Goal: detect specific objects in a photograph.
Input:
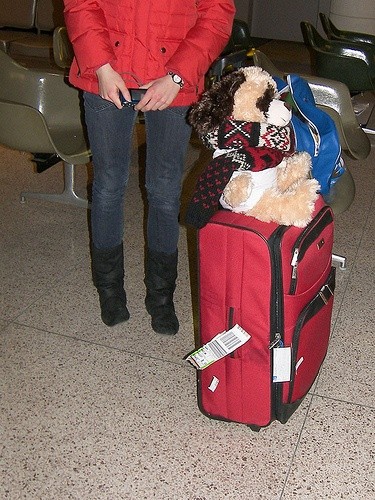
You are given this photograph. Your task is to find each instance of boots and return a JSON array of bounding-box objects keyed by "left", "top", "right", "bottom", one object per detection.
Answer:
[
  {"left": 89, "top": 243, "right": 129, "bottom": 327},
  {"left": 144, "top": 245, "right": 178, "bottom": 334}
]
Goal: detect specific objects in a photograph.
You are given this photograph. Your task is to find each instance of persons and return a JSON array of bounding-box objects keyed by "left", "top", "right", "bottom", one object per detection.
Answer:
[{"left": 61, "top": 0, "right": 238, "bottom": 336}]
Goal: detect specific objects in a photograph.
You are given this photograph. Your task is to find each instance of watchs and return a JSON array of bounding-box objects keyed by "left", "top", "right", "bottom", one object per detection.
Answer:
[{"left": 167, "top": 71, "right": 184, "bottom": 89}]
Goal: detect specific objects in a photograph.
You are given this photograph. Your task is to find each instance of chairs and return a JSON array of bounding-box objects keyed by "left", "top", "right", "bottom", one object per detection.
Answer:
[
  {"left": 8, "top": 0, "right": 64, "bottom": 59},
  {"left": 231, "top": 19, "right": 254, "bottom": 49},
  {"left": 319, "top": 12, "right": 375, "bottom": 51},
  {"left": 300, "top": 20, "right": 374, "bottom": 92},
  {"left": 0, "top": 47, "right": 93, "bottom": 210},
  {"left": 0, "top": 0, "right": 36, "bottom": 52},
  {"left": 328, "top": 161, "right": 357, "bottom": 272},
  {"left": 252, "top": 50, "right": 375, "bottom": 161}
]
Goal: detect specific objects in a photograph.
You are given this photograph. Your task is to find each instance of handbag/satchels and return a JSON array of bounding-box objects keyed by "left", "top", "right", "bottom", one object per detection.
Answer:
[{"left": 270, "top": 74, "right": 345, "bottom": 196}]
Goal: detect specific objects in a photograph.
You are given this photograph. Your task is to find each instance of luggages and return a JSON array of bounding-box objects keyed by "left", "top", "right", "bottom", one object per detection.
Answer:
[{"left": 188, "top": 48, "right": 335, "bottom": 433}]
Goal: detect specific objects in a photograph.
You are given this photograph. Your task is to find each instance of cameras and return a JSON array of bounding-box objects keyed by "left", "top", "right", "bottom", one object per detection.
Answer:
[{"left": 119, "top": 88, "right": 151, "bottom": 108}]
